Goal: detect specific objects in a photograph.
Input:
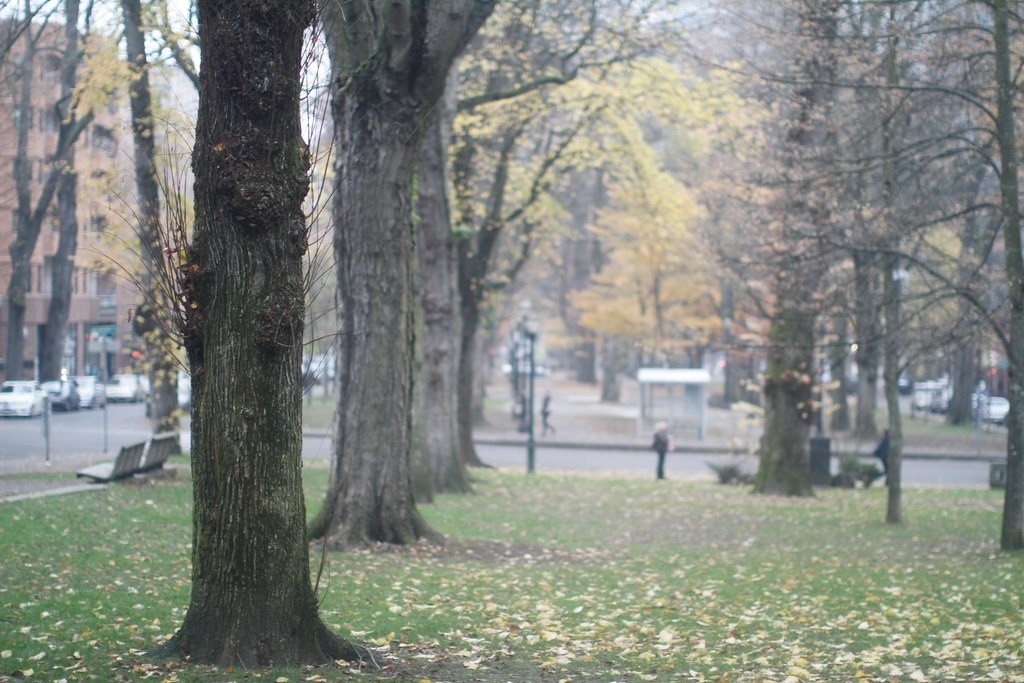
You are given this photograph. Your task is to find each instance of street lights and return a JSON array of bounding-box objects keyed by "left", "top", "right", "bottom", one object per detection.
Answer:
[{"left": 520, "top": 318, "right": 542, "bottom": 472}]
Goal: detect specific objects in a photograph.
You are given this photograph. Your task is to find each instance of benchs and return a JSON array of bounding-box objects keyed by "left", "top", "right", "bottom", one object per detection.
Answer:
[
  {"left": 136, "top": 434, "right": 176, "bottom": 473},
  {"left": 76, "top": 441, "right": 146, "bottom": 483}
]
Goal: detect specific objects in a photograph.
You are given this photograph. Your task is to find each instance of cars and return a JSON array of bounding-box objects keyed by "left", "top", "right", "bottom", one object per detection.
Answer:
[
  {"left": 0, "top": 380, "right": 50, "bottom": 417},
  {"left": 42, "top": 380, "right": 81, "bottom": 412},
  {"left": 105, "top": 373, "right": 146, "bottom": 404},
  {"left": 68, "top": 375, "right": 108, "bottom": 409},
  {"left": 898, "top": 374, "right": 1010, "bottom": 424}
]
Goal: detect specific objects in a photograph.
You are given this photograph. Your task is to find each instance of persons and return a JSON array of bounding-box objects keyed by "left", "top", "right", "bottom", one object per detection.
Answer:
[
  {"left": 541, "top": 392, "right": 556, "bottom": 437},
  {"left": 652, "top": 422, "right": 669, "bottom": 478},
  {"left": 873, "top": 429, "right": 890, "bottom": 484}
]
